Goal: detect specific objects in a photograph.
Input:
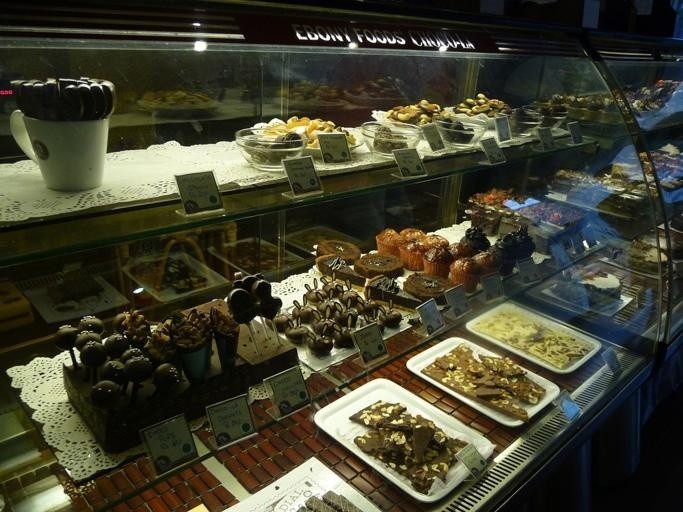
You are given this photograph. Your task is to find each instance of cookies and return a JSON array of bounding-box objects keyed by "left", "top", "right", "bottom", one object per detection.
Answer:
[
  {"left": 295, "top": 490, "right": 363, "bottom": 512},
  {"left": 257, "top": 91, "right": 516, "bottom": 147},
  {"left": 349, "top": 400, "right": 467, "bottom": 494},
  {"left": 136, "top": 76, "right": 418, "bottom": 108}
]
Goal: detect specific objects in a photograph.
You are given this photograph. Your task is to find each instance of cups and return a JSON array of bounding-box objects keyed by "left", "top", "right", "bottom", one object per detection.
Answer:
[{"left": 7, "top": 110, "right": 110, "bottom": 192}]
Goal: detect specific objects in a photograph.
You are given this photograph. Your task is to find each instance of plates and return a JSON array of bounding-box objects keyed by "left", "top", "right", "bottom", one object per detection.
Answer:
[
  {"left": 121, "top": 251, "right": 231, "bottom": 306},
  {"left": 279, "top": 226, "right": 365, "bottom": 266},
  {"left": 311, "top": 125, "right": 362, "bottom": 157},
  {"left": 381, "top": 104, "right": 508, "bottom": 129},
  {"left": 276, "top": 90, "right": 419, "bottom": 111},
  {"left": 463, "top": 301, "right": 601, "bottom": 375},
  {"left": 407, "top": 336, "right": 561, "bottom": 429},
  {"left": 136, "top": 101, "right": 224, "bottom": 116},
  {"left": 30, "top": 275, "right": 129, "bottom": 322},
  {"left": 208, "top": 237, "right": 308, "bottom": 283},
  {"left": 313, "top": 378, "right": 493, "bottom": 507}
]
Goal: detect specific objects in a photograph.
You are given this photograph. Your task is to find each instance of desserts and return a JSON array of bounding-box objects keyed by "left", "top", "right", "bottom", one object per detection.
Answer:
[{"left": 57, "top": 145, "right": 682, "bottom": 454}]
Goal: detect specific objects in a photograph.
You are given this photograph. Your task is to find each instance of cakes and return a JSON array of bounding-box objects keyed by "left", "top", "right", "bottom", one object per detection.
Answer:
[
  {"left": 0, "top": 280, "right": 35, "bottom": 336},
  {"left": 535, "top": 77, "right": 682, "bottom": 115}
]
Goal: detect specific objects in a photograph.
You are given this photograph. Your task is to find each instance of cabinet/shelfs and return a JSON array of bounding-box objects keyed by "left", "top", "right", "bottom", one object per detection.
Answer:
[{"left": 0, "top": 0, "right": 683, "bottom": 512}]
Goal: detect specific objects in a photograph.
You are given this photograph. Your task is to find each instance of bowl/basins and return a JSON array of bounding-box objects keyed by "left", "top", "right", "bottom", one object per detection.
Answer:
[
  {"left": 437, "top": 119, "right": 488, "bottom": 148},
  {"left": 234, "top": 128, "right": 308, "bottom": 175},
  {"left": 501, "top": 106, "right": 569, "bottom": 136},
  {"left": 362, "top": 123, "right": 422, "bottom": 157}
]
